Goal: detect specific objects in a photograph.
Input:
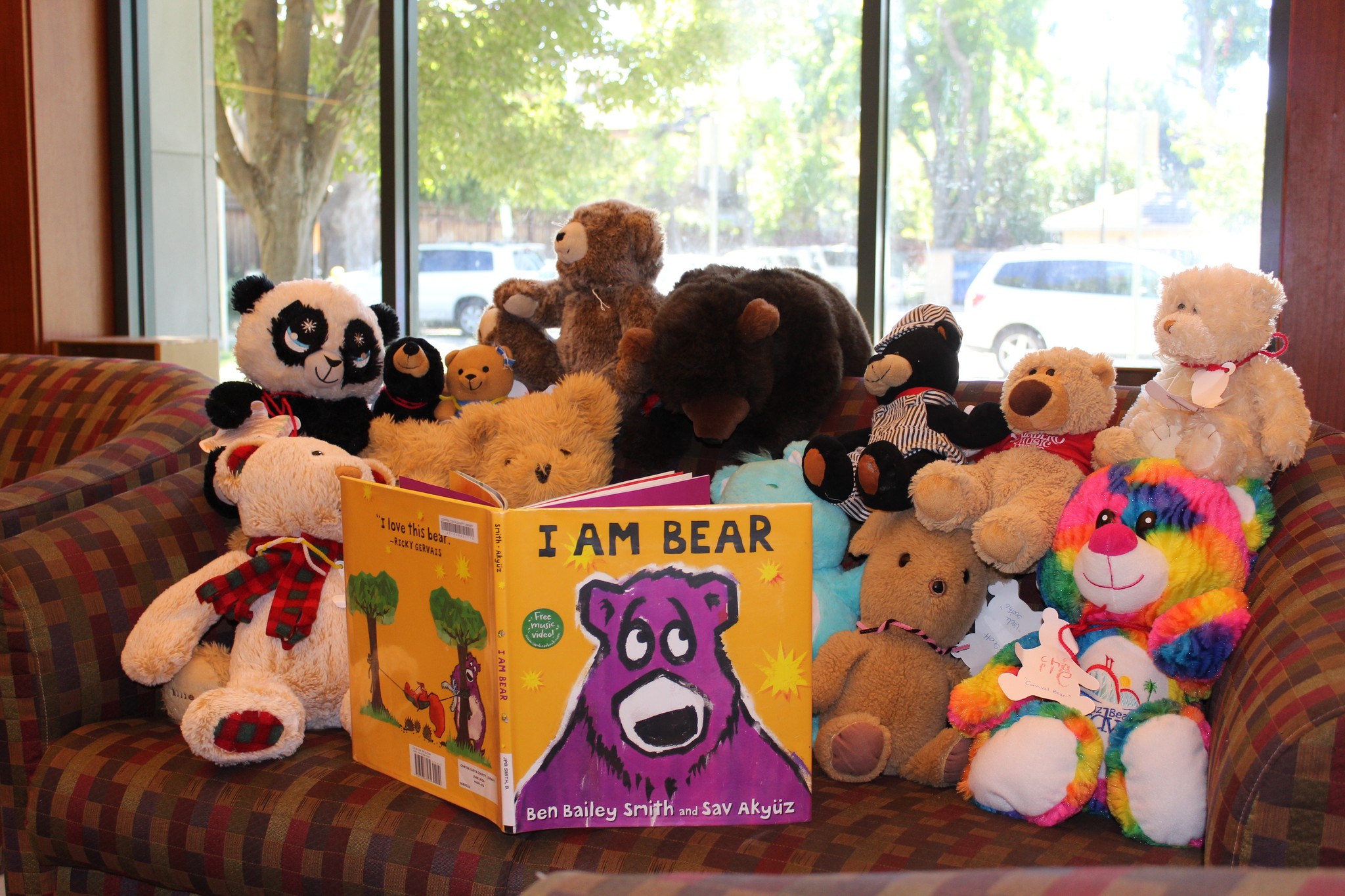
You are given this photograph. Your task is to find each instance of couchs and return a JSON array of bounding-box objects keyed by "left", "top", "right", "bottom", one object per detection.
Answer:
[{"left": 0, "top": 354, "right": 1345, "bottom": 896}]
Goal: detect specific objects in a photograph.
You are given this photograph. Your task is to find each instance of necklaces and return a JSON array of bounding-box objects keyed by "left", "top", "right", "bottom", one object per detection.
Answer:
[{"left": 1180, "top": 331, "right": 1288, "bottom": 406}]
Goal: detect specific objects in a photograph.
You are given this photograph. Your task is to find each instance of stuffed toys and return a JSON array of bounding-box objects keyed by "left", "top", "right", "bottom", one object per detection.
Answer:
[
  {"left": 907, "top": 347, "right": 1118, "bottom": 573},
  {"left": 201, "top": 275, "right": 399, "bottom": 528},
  {"left": 119, "top": 435, "right": 391, "bottom": 764},
  {"left": 1090, "top": 261, "right": 1312, "bottom": 486},
  {"left": 947, "top": 457, "right": 1278, "bottom": 846},
  {"left": 801, "top": 303, "right": 1012, "bottom": 521},
  {"left": 371, "top": 336, "right": 447, "bottom": 424},
  {"left": 434, "top": 345, "right": 514, "bottom": 425},
  {"left": 337, "top": 473, "right": 814, "bottom": 832},
  {"left": 811, "top": 508, "right": 1019, "bottom": 789},
  {"left": 608, "top": 266, "right": 876, "bottom": 486},
  {"left": 479, "top": 204, "right": 666, "bottom": 397},
  {"left": 366, "top": 369, "right": 626, "bottom": 509}
]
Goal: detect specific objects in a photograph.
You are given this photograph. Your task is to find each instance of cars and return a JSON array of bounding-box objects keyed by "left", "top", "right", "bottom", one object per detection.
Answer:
[
  {"left": 721, "top": 241, "right": 858, "bottom": 309},
  {"left": 961, "top": 252, "right": 1180, "bottom": 385}
]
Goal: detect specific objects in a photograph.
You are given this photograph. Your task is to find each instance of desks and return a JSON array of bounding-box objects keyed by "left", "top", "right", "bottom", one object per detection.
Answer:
[{"left": 51, "top": 336, "right": 219, "bottom": 382}]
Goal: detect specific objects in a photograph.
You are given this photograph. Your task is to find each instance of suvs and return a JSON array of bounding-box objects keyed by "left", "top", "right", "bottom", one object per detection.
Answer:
[{"left": 329, "top": 241, "right": 553, "bottom": 338}]
